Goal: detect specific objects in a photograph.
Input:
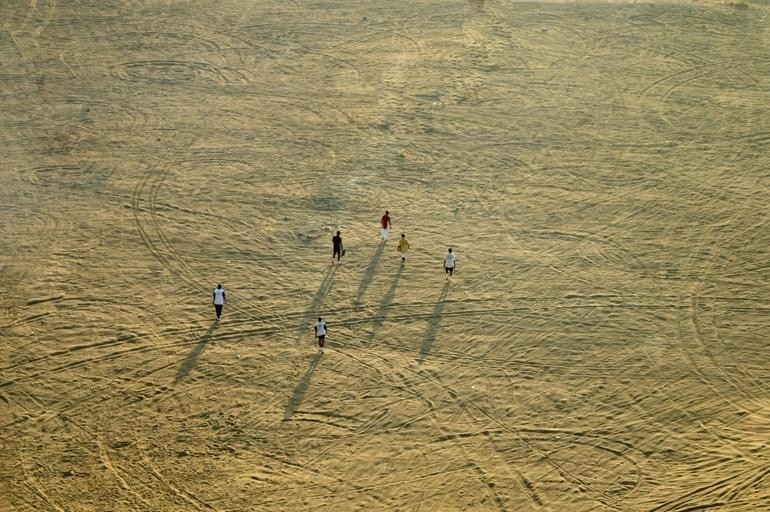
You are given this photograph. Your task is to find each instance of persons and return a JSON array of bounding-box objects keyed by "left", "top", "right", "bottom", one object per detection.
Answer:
[
  {"left": 331, "top": 230, "right": 343, "bottom": 265},
  {"left": 397, "top": 233, "right": 409, "bottom": 267},
  {"left": 443, "top": 247, "right": 456, "bottom": 283},
  {"left": 380, "top": 210, "right": 392, "bottom": 241},
  {"left": 314, "top": 316, "right": 328, "bottom": 354},
  {"left": 212, "top": 284, "right": 227, "bottom": 320}
]
